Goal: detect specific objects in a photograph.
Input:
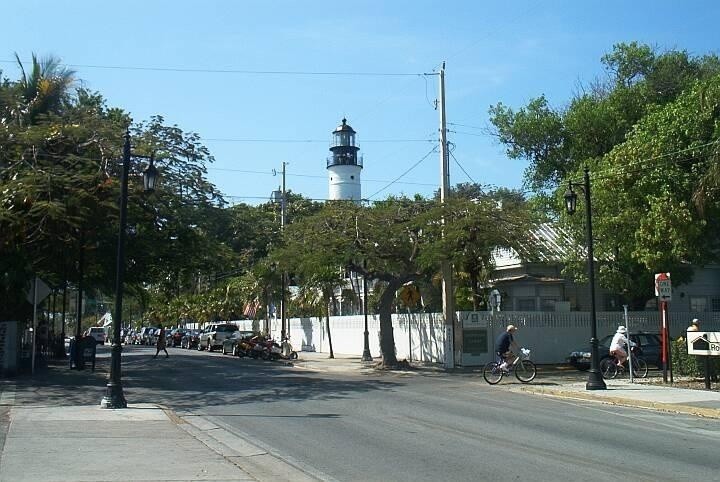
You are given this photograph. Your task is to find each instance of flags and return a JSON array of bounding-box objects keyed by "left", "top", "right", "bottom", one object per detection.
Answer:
[{"left": 243, "top": 298, "right": 258, "bottom": 321}]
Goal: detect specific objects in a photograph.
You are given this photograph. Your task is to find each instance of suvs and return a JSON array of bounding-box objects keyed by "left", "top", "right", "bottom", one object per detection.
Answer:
[{"left": 197, "top": 324, "right": 239, "bottom": 351}]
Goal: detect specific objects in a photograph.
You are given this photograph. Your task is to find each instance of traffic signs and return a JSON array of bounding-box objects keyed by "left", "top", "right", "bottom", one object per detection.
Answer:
[{"left": 655, "top": 273, "right": 670, "bottom": 296}]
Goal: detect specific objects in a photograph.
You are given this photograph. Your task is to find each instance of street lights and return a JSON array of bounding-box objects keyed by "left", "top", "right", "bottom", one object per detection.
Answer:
[
  {"left": 564, "top": 163, "right": 607, "bottom": 390},
  {"left": 101, "top": 127, "right": 160, "bottom": 409}
]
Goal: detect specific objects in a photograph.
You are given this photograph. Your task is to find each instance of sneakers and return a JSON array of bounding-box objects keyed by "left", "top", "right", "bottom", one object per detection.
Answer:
[
  {"left": 499, "top": 364, "right": 509, "bottom": 373},
  {"left": 617, "top": 363, "right": 624, "bottom": 367}
]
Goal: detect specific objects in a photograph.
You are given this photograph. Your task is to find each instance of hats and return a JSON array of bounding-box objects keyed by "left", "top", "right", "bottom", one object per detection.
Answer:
[
  {"left": 507, "top": 325, "right": 518, "bottom": 332},
  {"left": 693, "top": 319, "right": 702, "bottom": 325},
  {"left": 616, "top": 326, "right": 627, "bottom": 333}
]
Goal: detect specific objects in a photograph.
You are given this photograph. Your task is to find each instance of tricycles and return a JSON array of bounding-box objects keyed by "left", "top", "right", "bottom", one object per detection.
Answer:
[{"left": 70, "top": 337, "right": 96, "bottom": 370}]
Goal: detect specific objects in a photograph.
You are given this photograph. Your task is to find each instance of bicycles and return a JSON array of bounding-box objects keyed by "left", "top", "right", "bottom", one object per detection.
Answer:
[
  {"left": 599, "top": 345, "right": 648, "bottom": 379},
  {"left": 483, "top": 348, "right": 536, "bottom": 384}
]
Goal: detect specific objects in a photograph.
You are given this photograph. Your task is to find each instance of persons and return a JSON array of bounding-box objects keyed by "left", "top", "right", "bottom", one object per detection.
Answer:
[
  {"left": 153, "top": 324, "right": 170, "bottom": 359},
  {"left": 494, "top": 324, "right": 525, "bottom": 372},
  {"left": 610, "top": 326, "right": 639, "bottom": 367},
  {"left": 687, "top": 318, "right": 702, "bottom": 331}
]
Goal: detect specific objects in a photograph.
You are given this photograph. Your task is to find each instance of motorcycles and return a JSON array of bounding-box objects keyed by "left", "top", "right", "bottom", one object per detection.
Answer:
[{"left": 237, "top": 333, "right": 298, "bottom": 360}]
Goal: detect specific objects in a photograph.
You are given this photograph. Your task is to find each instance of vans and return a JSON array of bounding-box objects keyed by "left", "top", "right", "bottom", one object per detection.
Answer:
[{"left": 87, "top": 327, "right": 105, "bottom": 345}]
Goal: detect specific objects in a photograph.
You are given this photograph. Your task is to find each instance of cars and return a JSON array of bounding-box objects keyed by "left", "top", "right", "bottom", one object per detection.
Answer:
[
  {"left": 222, "top": 331, "right": 261, "bottom": 356},
  {"left": 570, "top": 331, "right": 675, "bottom": 371},
  {"left": 120, "top": 327, "right": 202, "bottom": 350}
]
